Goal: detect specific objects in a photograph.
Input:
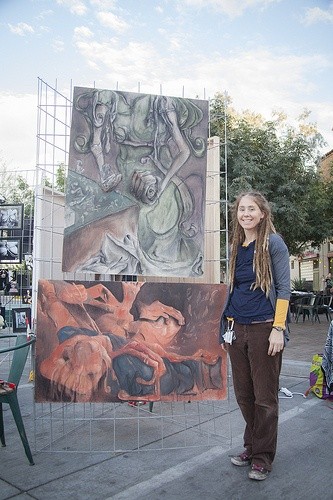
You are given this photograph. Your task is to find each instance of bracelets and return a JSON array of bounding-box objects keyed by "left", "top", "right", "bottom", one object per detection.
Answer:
[{"left": 272, "top": 326, "right": 283, "bottom": 331}]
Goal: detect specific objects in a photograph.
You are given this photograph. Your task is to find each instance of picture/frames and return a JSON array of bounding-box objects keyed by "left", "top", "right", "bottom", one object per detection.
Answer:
[
  {"left": 0, "top": 203, "right": 24, "bottom": 230},
  {"left": 0, "top": 236, "right": 23, "bottom": 264},
  {"left": 12, "top": 307, "right": 31, "bottom": 333}
]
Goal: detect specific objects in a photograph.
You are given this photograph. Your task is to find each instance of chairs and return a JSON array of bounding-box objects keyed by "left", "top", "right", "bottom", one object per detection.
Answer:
[
  {"left": 295, "top": 293, "right": 333, "bottom": 325},
  {"left": 0, "top": 333, "right": 36, "bottom": 465}
]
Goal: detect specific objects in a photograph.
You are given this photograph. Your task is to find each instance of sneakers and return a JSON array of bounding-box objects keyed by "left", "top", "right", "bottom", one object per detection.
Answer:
[
  {"left": 231, "top": 451, "right": 249, "bottom": 466},
  {"left": 249, "top": 463, "right": 268, "bottom": 480}
]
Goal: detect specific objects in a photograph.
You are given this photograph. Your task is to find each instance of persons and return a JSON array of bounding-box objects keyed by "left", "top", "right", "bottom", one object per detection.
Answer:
[{"left": 219, "top": 191, "right": 292, "bottom": 481}]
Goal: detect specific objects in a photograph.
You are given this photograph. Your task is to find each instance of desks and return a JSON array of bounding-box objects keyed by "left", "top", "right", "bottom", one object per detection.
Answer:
[{"left": 290, "top": 291, "right": 314, "bottom": 323}]
